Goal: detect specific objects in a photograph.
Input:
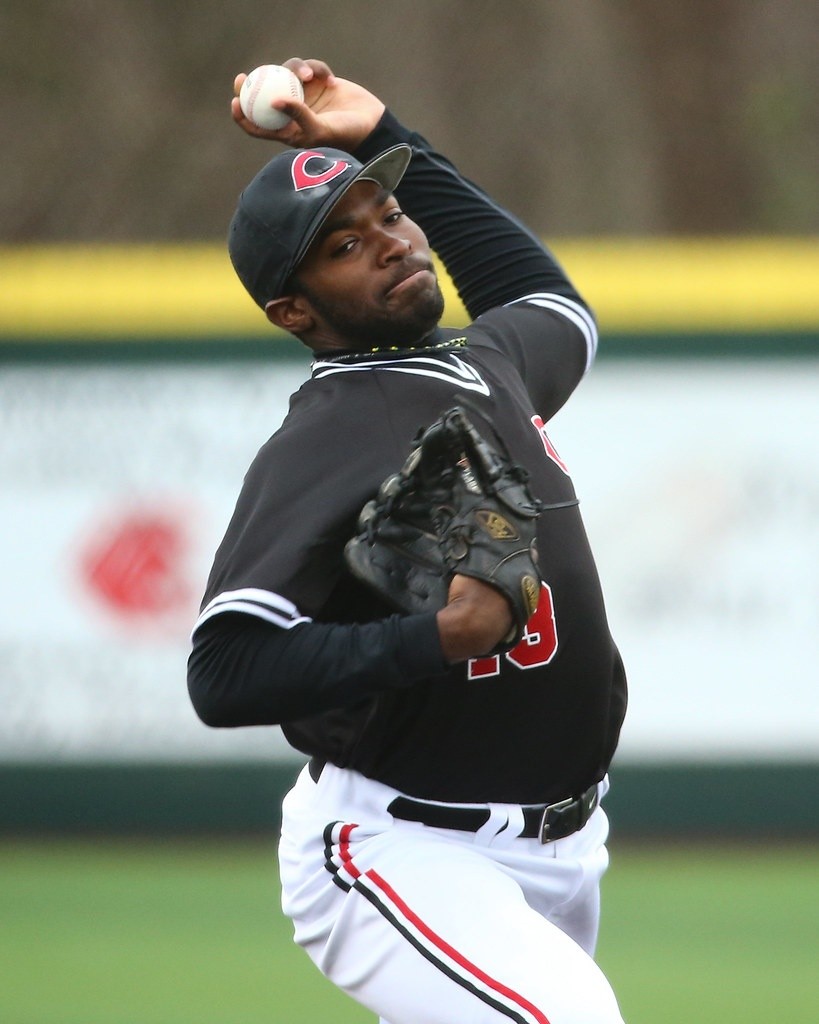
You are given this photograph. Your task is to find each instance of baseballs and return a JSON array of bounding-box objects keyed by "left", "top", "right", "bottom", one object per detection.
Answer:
[{"left": 239, "top": 63, "right": 302, "bottom": 130}]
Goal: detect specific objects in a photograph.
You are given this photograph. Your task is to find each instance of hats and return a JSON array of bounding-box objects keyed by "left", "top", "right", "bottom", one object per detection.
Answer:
[{"left": 225, "top": 141, "right": 412, "bottom": 313}]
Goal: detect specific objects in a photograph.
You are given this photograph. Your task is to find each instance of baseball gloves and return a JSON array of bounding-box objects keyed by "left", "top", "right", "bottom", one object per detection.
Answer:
[{"left": 340, "top": 408, "right": 545, "bottom": 655}]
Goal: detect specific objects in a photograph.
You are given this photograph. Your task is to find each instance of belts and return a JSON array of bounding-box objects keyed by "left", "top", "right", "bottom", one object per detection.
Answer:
[{"left": 308, "top": 755, "right": 601, "bottom": 844}]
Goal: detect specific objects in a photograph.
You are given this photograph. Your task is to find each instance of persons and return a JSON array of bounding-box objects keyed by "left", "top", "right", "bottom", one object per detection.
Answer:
[{"left": 185, "top": 58, "right": 623, "bottom": 1024}]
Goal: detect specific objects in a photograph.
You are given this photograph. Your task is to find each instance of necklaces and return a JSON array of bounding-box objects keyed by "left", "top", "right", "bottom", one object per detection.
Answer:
[{"left": 310, "top": 336, "right": 468, "bottom": 367}]
